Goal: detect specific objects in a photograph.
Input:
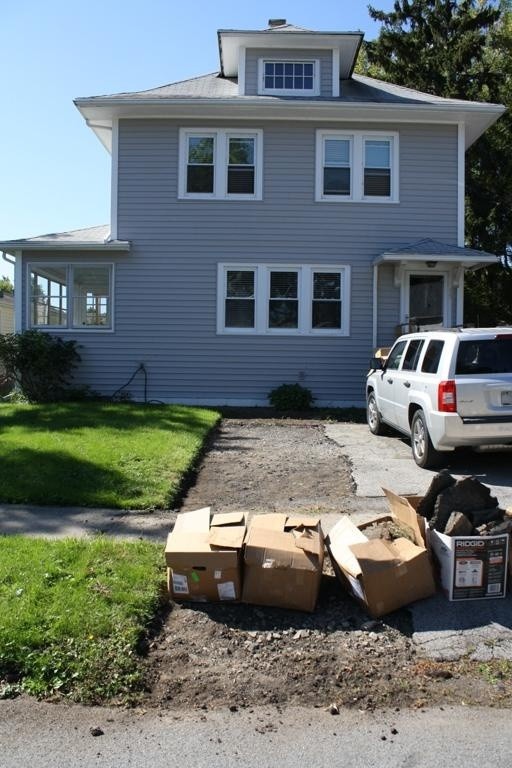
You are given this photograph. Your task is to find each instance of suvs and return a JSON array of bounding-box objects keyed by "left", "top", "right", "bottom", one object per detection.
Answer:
[{"left": 365, "top": 328, "right": 512, "bottom": 468}]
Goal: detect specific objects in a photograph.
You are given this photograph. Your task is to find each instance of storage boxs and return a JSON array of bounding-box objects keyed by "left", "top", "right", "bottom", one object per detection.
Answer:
[
  {"left": 321, "top": 488, "right": 435, "bottom": 619},
  {"left": 241, "top": 511, "right": 324, "bottom": 613},
  {"left": 417, "top": 468, "right": 509, "bottom": 601},
  {"left": 165, "top": 506, "right": 242, "bottom": 603}
]
